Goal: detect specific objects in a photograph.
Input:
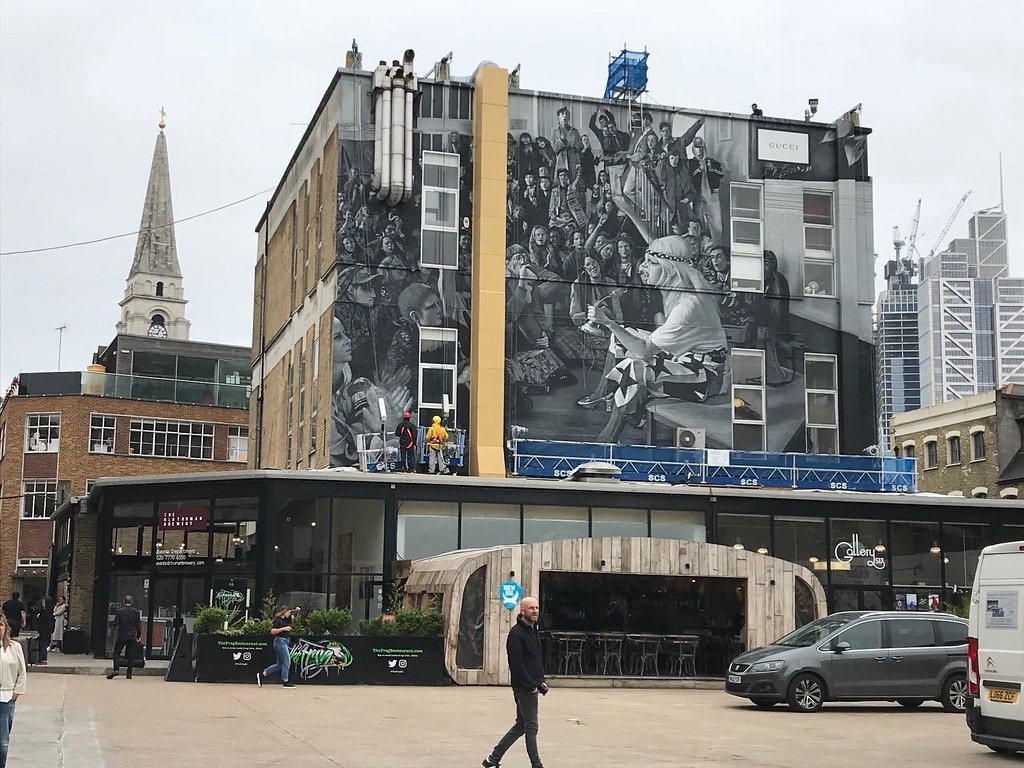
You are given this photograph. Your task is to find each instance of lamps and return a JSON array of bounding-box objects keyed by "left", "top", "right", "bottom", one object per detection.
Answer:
[
  {"left": 930, "top": 541, "right": 950, "bottom": 565},
  {"left": 875, "top": 538, "right": 886, "bottom": 552},
  {"left": 758, "top": 542, "right": 769, "bottom": 555},
  {"left": 733, "top": 537, "right": 744, "bottom": 550},
  {"left": 804, "top": 97, "right": 818, "bottom": 121}
]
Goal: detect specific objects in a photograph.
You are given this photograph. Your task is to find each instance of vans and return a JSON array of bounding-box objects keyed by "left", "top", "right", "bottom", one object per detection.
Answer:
[{"left": 965, "top": 540, "right": 1024, "bottom": 755}]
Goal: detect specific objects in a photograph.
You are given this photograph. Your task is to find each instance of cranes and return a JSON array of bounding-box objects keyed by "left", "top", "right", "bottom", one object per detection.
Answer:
[
  {"left": 908, "top": 198, "right": 922, "bottom": 260},
  {"left": 925, "top": 190, "right": 974, "bottom": 258}
]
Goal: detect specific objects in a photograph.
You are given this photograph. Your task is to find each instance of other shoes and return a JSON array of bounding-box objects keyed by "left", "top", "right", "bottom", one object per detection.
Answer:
[
  {"left": 35, "top": 662, "right": 48, "bottom": 667},
  {"left": 126, "top": 674, "right": 132, "bottom": 679},
  {"left": 106, "top": 670, "right": 119, "bottom": 679}
]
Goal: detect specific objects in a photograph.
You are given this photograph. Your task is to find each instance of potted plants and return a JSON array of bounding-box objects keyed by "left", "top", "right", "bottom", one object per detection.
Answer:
[{"left": 192, "top": 572, "right": 448, "bottom": 686}]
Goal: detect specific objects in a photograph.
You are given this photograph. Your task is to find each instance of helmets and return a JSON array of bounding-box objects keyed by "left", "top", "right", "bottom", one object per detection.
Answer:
[
  {"left": 432, "top": 416, "right": 441, "bottom": 423},
  {"left": 403, "top": 412, "right": 411, "bottom": 418}
]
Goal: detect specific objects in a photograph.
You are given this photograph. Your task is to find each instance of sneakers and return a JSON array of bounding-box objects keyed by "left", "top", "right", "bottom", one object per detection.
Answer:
[
  {"left": 257, "top": 673, "right": 264, "bottom": 687},
  {"left": 481, "top": 757, "right": 500, "bottom": 768},
  {"left": 282, "top": 682, "right": 296, "bottom": 689}
]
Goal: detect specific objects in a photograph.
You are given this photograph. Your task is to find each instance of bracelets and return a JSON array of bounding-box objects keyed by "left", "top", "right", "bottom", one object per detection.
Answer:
[{"left": 22, "top": 620, "right": 26, "bottom": 622}]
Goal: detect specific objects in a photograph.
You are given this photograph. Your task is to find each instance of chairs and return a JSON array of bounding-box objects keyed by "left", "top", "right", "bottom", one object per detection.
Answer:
[{"left": 558, "top": 635, "right": 744, "bottom": 677}]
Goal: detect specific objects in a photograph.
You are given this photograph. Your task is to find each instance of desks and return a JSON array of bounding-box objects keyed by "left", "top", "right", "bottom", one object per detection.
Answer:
[
  {"left": 597, "top": 638, "right": 621, "bottom": 675},
  {"left": 559, "top": 636, "right": 585, "bottom": 673},
  {"left": 634, "top": 639, "right": 662, "bottom": 674},
  {"left": 664, "top": 638, "right": 700, "bottom": 674}
]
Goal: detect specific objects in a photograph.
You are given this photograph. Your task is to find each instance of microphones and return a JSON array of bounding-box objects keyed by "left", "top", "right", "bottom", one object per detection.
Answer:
[{"left": 580, "top": 287, "right": 626, "bottom": 336}]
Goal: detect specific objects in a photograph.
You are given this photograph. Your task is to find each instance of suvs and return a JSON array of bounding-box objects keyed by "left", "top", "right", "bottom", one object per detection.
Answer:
[{"left": 724, "top": 610, "right": 969, "bottom": 713}]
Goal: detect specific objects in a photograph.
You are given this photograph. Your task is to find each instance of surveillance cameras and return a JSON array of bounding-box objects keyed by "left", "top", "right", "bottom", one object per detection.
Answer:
[{"left": 867, "top": 447, "right": 879, "bottom": 455}]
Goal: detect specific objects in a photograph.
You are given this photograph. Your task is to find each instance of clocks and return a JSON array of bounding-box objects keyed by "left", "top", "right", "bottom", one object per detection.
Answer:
[{"left": 147, "top": 324, "right": 168, "bottom": 339}]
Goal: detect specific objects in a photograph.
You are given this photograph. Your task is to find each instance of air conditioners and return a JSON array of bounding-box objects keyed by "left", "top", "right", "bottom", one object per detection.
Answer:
[{"left": 673, "top": 426, "right": 705, "bottom": 447}]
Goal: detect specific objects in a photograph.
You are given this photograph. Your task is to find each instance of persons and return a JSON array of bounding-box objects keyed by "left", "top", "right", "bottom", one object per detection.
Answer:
[
  {"left": 2, "top": 590, "right": 26, "bottom": 637},
  {"left": 507, "top": 103, "right": 790, "bottom": 446},
  {"left": 482, "top": 597, "right": 551, "bottom": 768},
  {"left": 46, "top": 594, "right": 69, "bottom": 653},
  {"left": 751, "top": 104, "right": 763, "bottom": 116},
  {"left": 118, "top": 635, "right": 147, "bottom": 668},
  {"left": 106, "top": 595, "right": 142, "bottom": 680},
  {"left": 256, "top": 605, "right": 301, "bottom": 688},
  {"left": 330, "top": 131, "right": 474, "bottom": 474},
  {"left": 424, "top": 416, "right": 451, "bottom": 474},
  {"left": 0, "top": 613, "right": 26, "bottom": 768},
  {"left": 395, "top": 412, "right": 418, "bottom": 473},
  {"left": 35, "top": 596, "right": 55, "bottom": 665}
]
